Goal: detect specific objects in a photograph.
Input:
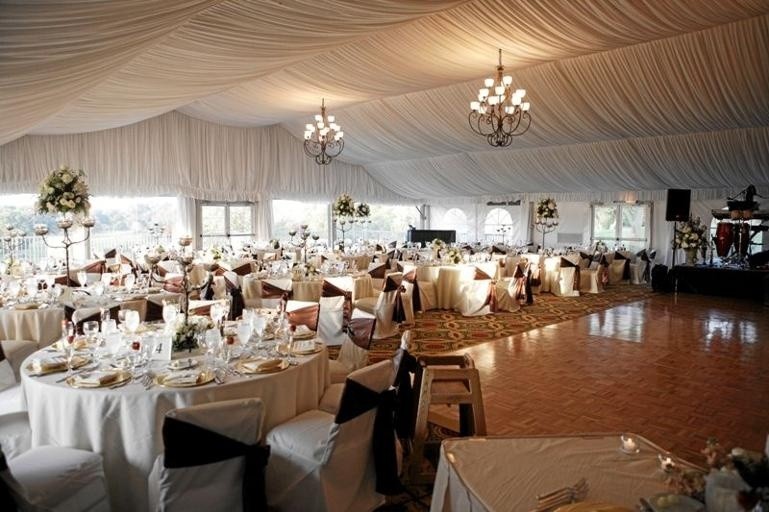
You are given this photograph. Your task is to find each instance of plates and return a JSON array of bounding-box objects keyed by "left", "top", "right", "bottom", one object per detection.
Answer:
[
  {"left": 29, "top": 300, "right": 323, "bottom": 388},
  {"left": 648, "top": 492, "right": 704, "bottom": 511}
]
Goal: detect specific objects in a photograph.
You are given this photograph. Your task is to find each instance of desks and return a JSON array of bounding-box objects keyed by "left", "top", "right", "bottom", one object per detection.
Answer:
[{"left": 429, "top": 430, "right": 703, "bottom": 512}]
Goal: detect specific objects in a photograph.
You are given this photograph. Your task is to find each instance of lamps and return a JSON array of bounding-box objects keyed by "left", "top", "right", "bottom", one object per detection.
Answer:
[
  {"left": 303, "top": 97, "right": 344, "bottom": 165},
  {"left": 35, "top": 215, "right": 95, "bottom": 295},
  {"left": 332, "top": 192, "right": 371, "bottom": 219},
  {"left": 468, "top": 49, "right": 530, "bottom": 149}
]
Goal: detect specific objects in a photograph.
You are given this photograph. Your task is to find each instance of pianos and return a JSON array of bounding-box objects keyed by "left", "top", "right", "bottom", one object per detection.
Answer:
[{"left": 711, "top": 209, "right": 769, "bottom": 220}]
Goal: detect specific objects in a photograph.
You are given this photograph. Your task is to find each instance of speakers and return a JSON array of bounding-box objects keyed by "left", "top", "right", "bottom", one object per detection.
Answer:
[
  {"left": 653, "top": 274, "right": 673, "bottom": 291},
  {"left": 751, "top": 251, "right": 769, "bottom": 266},
  {"left": 666, "top": 189, "right": 691, "bottom": 221}
]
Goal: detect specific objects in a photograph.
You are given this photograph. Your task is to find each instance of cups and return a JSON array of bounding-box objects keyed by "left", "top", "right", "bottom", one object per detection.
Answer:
[
  {"left": 705, "top": 470, "right": 744, "bottom": 511},
  {"left": 620, "top": 433, "right": 640, "bottom": 452},
  {"left": 658, "top": 453, "right": 677, "bottom": 472}
]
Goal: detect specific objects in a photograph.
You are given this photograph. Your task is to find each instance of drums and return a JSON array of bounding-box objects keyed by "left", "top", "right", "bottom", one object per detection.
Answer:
[
  {"left": 734, "top": 223, "right": 750, "bottom": 257},
  {"left": 716, "top": 223, "right": 734, "bottom": 257}
]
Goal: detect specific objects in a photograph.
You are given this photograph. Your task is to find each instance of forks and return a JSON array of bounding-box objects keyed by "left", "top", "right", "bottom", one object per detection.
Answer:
[{"left": 533, "top": 477, "right": 591, "bottom": 510}]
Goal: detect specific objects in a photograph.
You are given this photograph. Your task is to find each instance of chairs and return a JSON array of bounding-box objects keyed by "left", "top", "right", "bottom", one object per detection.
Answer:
[
  {"left": 1, "top": 439, "right": 106, "bottom": 512},
  {"left": 1, "top": 238, "right": 660, "bottom": 361},
  {"left": 145, "top": 398, "right": 275, "bottom": 512},
  {"left": 327, "top": 314, "right": 377, "bottom": 382},
  {"left": 320, "top": 328, "right": 413, "bottom": 415},
  {"left": 266, "top": 358, "right": 396, "bottom": 511},
  {"left": 1, "top": 362, "right": 30, "bottom": 442}
]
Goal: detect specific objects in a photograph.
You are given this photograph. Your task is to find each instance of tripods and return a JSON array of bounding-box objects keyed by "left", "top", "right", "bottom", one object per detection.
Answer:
[{"left": 725, "top": 223, "right": 749, "bottom": 268}]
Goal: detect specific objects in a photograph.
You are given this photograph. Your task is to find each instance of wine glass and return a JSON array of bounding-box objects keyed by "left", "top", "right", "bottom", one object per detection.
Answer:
[{"left": 0, "top": 269, "right": 150, "bottom": 304}]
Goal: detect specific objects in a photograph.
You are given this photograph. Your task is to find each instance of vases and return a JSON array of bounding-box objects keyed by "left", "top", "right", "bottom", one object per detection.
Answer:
[{"left": 683, "top": 247, "right": 696, "bottom": 266}]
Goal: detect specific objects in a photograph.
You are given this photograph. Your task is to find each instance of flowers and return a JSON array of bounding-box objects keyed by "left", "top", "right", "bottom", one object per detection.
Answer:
[
  {"left": 658, "top": 437, "right": 769, "bottom": 511},
  {"left": 35, "top": 168, "right": 90, "bottom": 218},
  {"left": 670, "top": 213, "right": 707, "bottom": 248},
  {"left": 536, "top": 198, "right": 559, "bottom": 218}
]
